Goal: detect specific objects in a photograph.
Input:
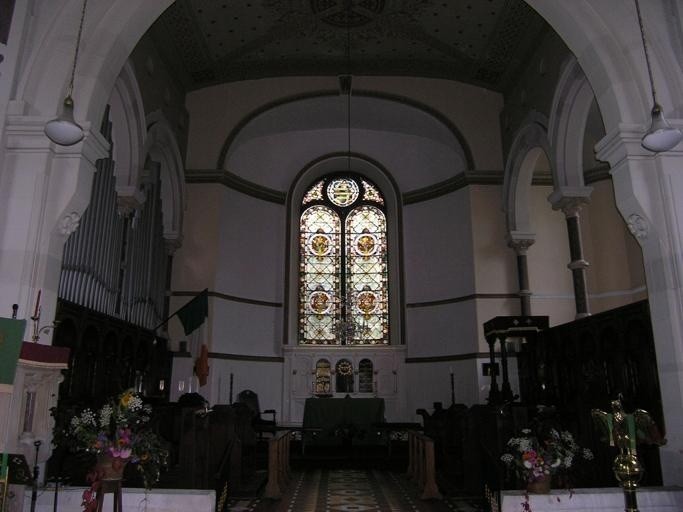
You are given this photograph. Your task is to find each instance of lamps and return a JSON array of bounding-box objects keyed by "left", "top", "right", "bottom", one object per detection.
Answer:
[
  {"left": 632, "top": 0, "right": 683, "bottom": 157},
  {"left": 43, "top": 2, "right": 85, "bottom": 146}
]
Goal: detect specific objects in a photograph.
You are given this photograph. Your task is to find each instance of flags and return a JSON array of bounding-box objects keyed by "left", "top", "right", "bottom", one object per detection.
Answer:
[
  {"left": 176, "top": 288, "right": 208, "bottom": 336},
  {"left": 0, "top": 317, "right": 26, "bottom": 394}
]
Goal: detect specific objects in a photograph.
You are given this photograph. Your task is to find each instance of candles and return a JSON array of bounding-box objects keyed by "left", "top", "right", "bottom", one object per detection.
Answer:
[{"left": 449, "top": 365, "right": 453, "bottom": 374}]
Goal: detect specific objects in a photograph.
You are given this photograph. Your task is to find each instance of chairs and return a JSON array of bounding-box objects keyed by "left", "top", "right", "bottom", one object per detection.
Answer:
[{"left": 238, "top": 389, "right": 276, "bottom": 425}]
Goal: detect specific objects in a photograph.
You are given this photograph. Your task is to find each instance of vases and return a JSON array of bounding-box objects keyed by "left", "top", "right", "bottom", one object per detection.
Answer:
[
  {"left": 95, "top": 454, "right": 131, "bottom": 482},
  {"left": 526, "top": 471, "right": 552, "bottom": 494}
]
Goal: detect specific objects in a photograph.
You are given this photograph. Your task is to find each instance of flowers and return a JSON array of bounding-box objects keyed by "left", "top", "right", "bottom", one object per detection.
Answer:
[
  {"left": 52, "top": 386, "right": 171, "bottom": 512},
  {"left": 498, "top": 424, "right": 593, "bottom": 512}
]
